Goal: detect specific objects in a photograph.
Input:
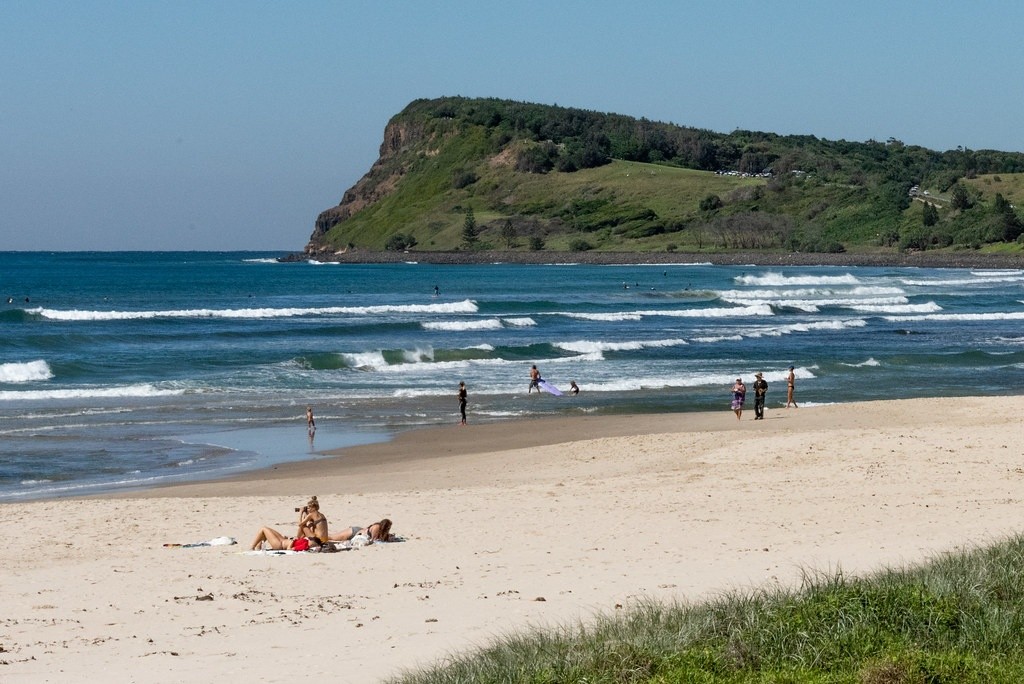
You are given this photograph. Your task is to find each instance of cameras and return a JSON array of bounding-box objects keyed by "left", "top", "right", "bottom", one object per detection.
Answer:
[{"left": 295, "top": 506, "right": 308, "bottom": 512}]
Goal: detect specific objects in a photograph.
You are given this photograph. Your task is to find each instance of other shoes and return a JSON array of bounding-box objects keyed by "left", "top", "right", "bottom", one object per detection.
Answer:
[{"left": 457, "top": 422, "right": 464, "bottom": 426}]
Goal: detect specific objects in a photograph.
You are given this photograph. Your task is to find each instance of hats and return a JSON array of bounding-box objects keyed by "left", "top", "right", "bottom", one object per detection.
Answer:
[
  {"left": 459, "top": 381, "right": 465, "bottom": 385},
  {"left": 755, "top": 373, "right": 763, "bottom": 378},
  {"left": 789, "top": 366, "right": 794, "bottom": 369}
]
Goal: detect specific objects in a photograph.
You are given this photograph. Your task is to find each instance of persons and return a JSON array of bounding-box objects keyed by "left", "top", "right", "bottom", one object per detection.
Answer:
[
  {"left": 328, "top": 519, "right": 394, "bottom": 542},
  {"left": 434, "top": 285, "right": 439, "bottom": 296},
  {"left": 751, "top": 372, "right": 768, "bottom": 420},
  {"left": 783, "top": 365, "right": 798, "bottom": 408},
  {"left": 569, "top": 380, "right": 579, "bottom": 396},
  {"left": 731, "top": 378, "right": 746, "bottom": 420},
  {"left": 458, "top": 380, "right": 468, "bottom": 426},
  {"left": 249, "top": 527, "right": 323, "bottom": 550},
  {"left": 307, "top": 429, "right": 315, "bottom": 448},
  {"left": 297, "top": 495, "right": 328, "bottom": 543},
  {"left": 528, "top": 364, "right": 541, "bottom": 393},
  {"left": 306, "top": 407, "right": 316, "bottom": 429}
]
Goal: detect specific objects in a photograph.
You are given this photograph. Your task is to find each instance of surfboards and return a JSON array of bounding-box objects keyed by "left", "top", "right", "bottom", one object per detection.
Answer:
[{"left": 537, "top": 378, "right": 562, "bottom": 395}]
